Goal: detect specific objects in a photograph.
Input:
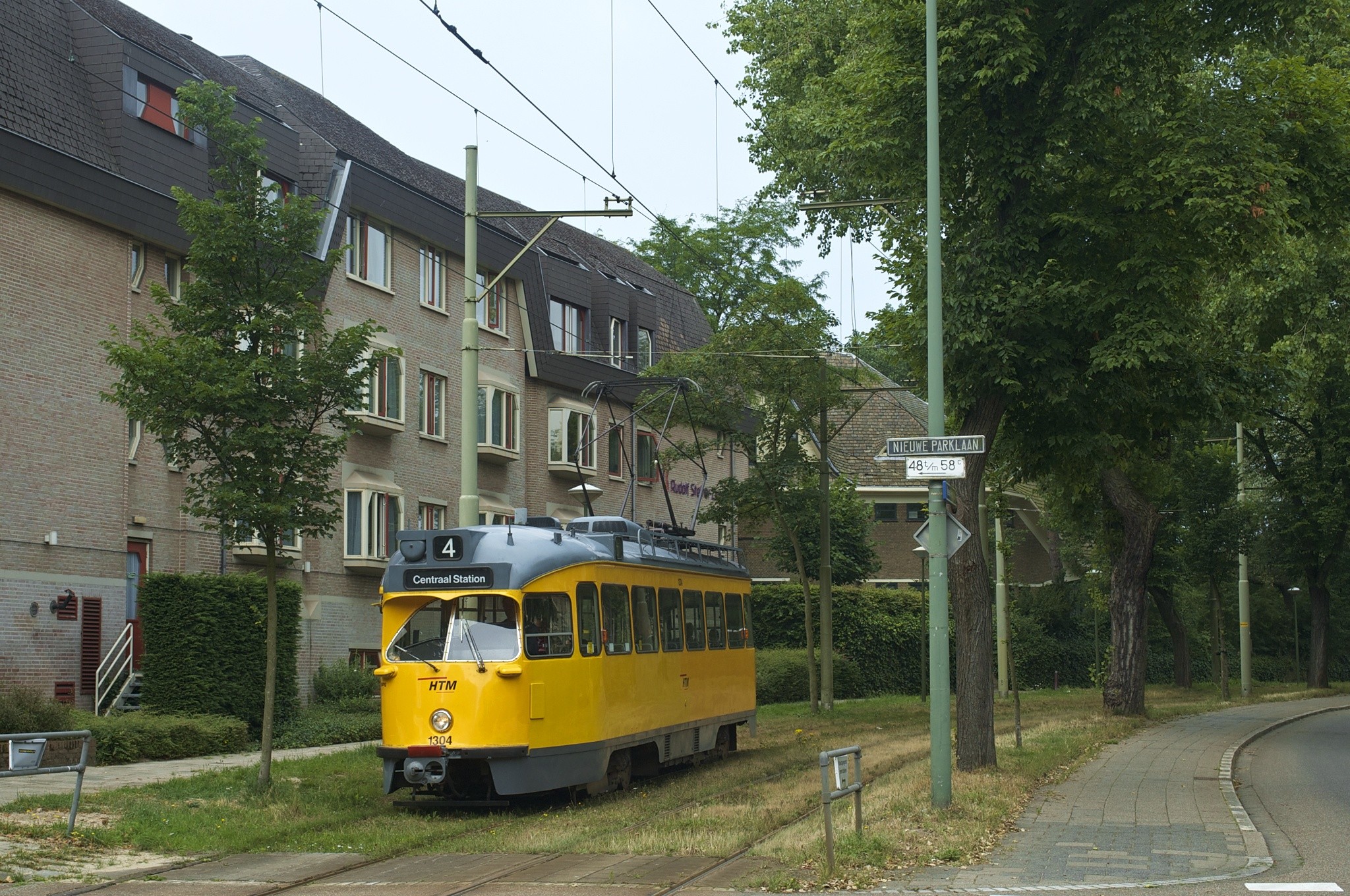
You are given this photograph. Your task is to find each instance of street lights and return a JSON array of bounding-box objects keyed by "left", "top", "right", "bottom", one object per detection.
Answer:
[
  {"left": 912, "top": 545, "right": 929, "bottom": 703},
  {"left": 779, "top": 356, "right": 836, "bottom": 711},
  {"left": 1287, "top": 586, "right": 1301, "bottom": 683}
]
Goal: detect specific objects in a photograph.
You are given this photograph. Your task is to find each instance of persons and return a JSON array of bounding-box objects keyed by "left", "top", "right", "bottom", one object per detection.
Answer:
[
  {"left": 685, "top": 622, "right": 697, "bottom": 649},
  {"left": 493, "top": 597, "right": 539, "bottom": 656},
  {"left": 709, "top": 629, "right": 721, "bottom": 648}
]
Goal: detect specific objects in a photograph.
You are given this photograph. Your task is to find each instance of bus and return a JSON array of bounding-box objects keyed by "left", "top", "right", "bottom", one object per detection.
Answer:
[{"left": 371, "top": 356, "right": 756, "bottom": 811}]
